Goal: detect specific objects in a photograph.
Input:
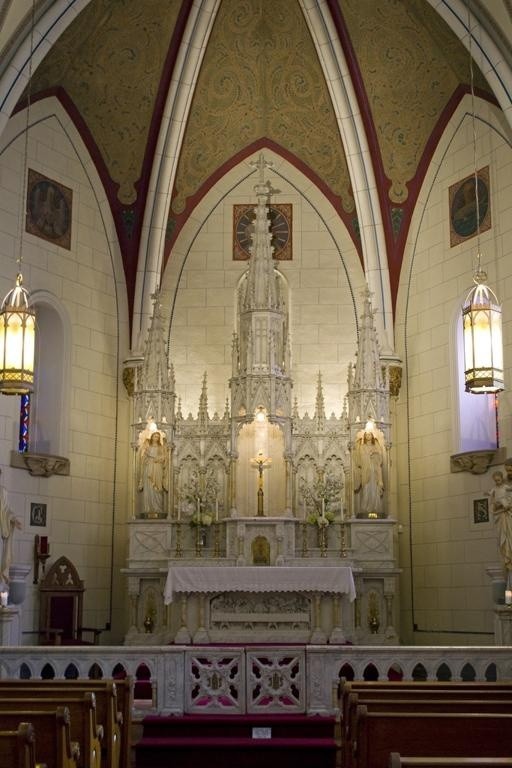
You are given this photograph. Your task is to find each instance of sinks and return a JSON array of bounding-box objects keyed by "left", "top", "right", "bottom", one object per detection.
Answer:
[{"left": 329, "top": 675, "right": 512, "bottom": 768}]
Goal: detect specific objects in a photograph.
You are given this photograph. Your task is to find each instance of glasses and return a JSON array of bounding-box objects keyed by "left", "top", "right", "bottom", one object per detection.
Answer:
[
  {"left": 0, "top": 1, "right": 39, "bottom": 396},
  {"left": 462, "top": 1, "right": 506, "bottom": 396}
]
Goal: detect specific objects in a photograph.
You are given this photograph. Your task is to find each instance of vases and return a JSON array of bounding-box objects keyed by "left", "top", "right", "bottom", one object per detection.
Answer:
[
  {"left": 307, "top": 491, "right": 336, "bottom": 530},
  {"left": 253, "top": 450, "right": 272, "bottom": 487},
  {"left": 179, "top": 490, "right": 216, "bottom": 527}
]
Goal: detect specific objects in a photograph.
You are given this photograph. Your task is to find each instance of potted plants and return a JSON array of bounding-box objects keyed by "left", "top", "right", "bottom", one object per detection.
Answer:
[
  {"left": 29, "top": 502, "right": 47, "bottom": 527},
  {"left": 473, "top": 498, "right": 490, "bottom": 525}
]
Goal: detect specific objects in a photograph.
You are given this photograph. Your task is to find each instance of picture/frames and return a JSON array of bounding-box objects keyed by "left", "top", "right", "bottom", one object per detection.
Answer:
[{"left": 165, "top": 566, "right": 356, "bottom": 646}]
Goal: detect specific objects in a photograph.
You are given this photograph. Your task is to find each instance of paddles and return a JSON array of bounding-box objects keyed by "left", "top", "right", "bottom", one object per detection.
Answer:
[{"left": 131, "top": 714, "right": 338, "bottom": 767}]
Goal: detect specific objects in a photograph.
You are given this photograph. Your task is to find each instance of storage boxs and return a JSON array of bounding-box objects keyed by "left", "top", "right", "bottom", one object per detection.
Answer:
[{"left": 0, "top": 675, "right": 146, "bottom": 767}]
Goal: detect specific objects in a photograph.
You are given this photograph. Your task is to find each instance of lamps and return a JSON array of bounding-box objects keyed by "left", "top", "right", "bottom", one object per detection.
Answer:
[{"left": 38, "top": 555, "right": 103, "bottom": 645}]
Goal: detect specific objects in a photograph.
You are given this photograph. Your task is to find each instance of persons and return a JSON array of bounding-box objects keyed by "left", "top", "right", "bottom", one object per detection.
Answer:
[
  {"left": 484, "top": 471, "right": 512, "bottom": 511},
  {"left": 355, "top": 432, "right": 384, "bottom": 514},
  {"left": 491, "top": 458, "right": 512, "bottom": 591},
  {"left": 138, "top": 432, "right": 169, "bottom": 519}
]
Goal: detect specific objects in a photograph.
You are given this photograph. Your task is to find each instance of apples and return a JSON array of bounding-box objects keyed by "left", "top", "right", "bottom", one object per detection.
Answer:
[
  {"left": 215, "top": 499, "right": 219, "bottom": 521},
  {"left": 303, "top": 501, "right": 307, "bottom": 522},
  {"left": 321, "top": 499, "right": 326, "bottom": 519},
  {"left": 196, "top": 499, "right": 201, "bottom": 522},
  {"left": 177, "top": 500, "right": 181, "bottom": 521},
  {"left": 340, "top": 499, "right": 345, "bottom": 522}
]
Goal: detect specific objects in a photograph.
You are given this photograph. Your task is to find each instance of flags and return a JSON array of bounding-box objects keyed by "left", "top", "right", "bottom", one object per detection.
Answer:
[
  {"left": 316, "top": 526, "right": 329, "bottom": 548},
  {"left": 196, "top": 524, "right": 206, "bottom": 547}
]
